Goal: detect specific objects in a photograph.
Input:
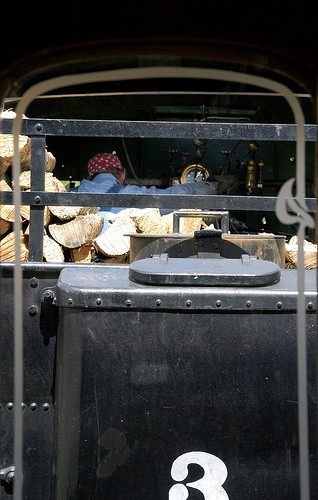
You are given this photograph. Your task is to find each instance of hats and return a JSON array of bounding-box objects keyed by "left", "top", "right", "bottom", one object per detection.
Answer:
[{"left": 87, "top": 150, "right": 123, "bottom": 178}]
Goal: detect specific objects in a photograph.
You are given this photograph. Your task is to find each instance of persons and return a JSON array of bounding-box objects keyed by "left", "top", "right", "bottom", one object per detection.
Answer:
[{"left": 70, "top": 152, "right": 219, "bottom": 251}]
